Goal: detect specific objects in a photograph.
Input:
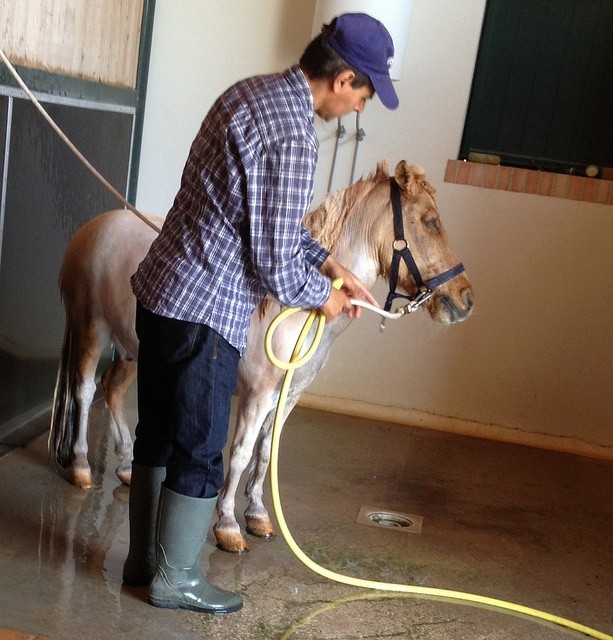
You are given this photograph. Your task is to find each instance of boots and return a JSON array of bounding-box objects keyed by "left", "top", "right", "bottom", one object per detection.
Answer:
[
  {"left": 148, "top": 482, "right": 244, "bottom": 614},
  {"left": 122, "top": 466, "right": 166, "bottom": 587}
]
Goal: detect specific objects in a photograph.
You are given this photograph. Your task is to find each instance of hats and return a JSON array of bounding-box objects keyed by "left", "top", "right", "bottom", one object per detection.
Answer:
[{"left": 321, "top": 13, "right": 399, "bottom": 110}]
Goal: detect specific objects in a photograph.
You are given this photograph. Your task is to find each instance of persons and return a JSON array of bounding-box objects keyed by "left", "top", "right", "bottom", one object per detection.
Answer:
[{"left": 122, "top": 13, "right": 399, "bottom": 615}]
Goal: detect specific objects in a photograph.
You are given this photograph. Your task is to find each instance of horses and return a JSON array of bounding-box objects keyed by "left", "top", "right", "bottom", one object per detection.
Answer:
[{"left": 47, "top": 159, "right": 475, "bottom": 553}]
